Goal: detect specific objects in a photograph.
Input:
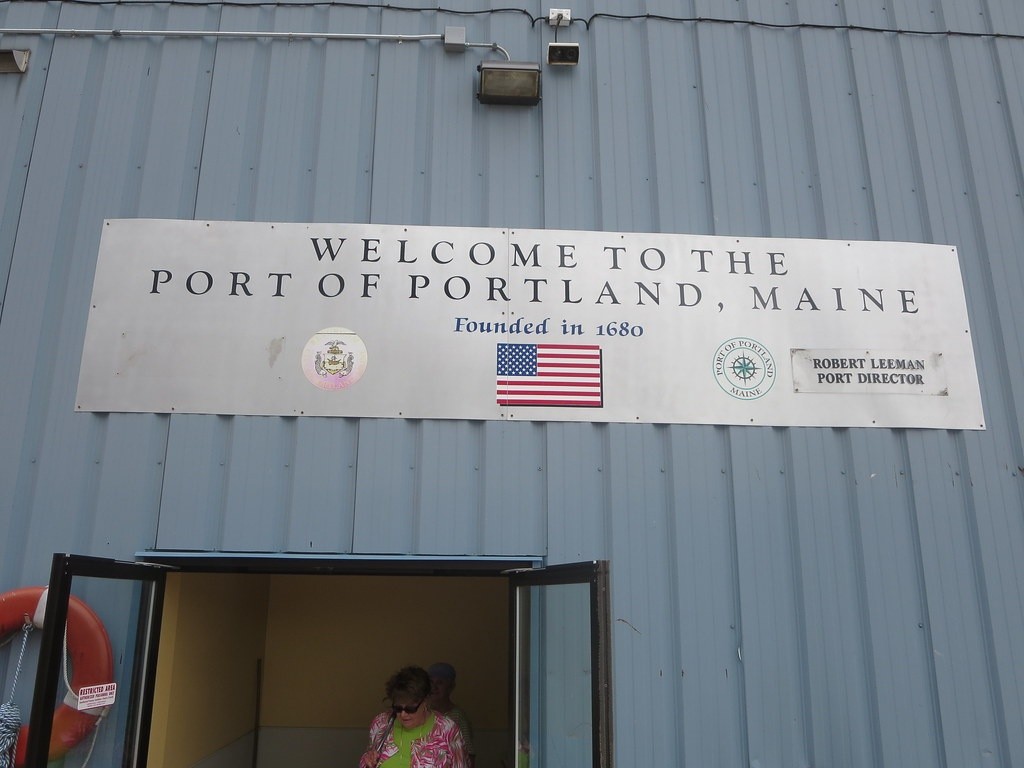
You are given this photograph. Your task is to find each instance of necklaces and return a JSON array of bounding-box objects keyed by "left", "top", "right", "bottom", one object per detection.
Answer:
[{"left": 398, "top": 724, "right": 424, "bottom": 759}]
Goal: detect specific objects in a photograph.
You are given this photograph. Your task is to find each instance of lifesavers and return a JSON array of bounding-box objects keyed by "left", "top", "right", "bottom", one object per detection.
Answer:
[{"left": 0, "top": 589, "right": 116, "bottom": 768}]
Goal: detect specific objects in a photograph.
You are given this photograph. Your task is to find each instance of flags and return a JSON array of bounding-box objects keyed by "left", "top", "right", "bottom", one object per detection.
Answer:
[{"left": 496, "top": 344, "right": 603, "bottom": 407}]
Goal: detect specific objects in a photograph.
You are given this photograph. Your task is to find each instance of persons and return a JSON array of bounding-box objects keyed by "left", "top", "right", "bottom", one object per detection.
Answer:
[
  {"left": 429, "top": 663, "right": 475, "bottom": 768},
  {"left": 357, "top": 665, "right": 466, "bottom": 768}
]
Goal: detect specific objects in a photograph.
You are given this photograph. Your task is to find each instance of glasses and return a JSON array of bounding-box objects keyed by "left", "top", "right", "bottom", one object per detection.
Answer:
[{"left": 390, "top": 697, "right": 426, "bottom": 713}]
